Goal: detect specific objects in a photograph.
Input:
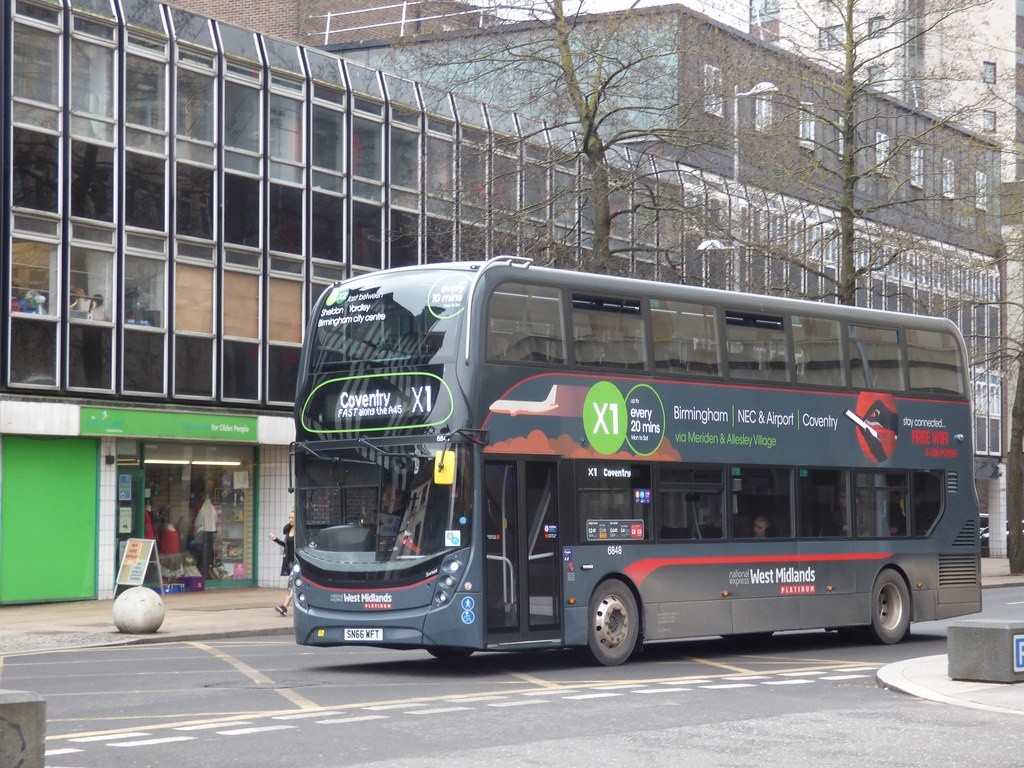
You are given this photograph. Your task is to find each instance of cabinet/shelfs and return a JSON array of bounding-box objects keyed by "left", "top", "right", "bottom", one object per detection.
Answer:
[{"left": 216, "top": 503, "right": 244, "bottom": 579}]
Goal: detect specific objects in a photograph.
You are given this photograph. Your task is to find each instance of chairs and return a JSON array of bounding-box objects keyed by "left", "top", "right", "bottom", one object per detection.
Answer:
[
  {"left": 488, "top": 326, "right": 936, "bottom": 393},
  {"left": 659, "top": 500, "right": 941, "bottom": 538}
]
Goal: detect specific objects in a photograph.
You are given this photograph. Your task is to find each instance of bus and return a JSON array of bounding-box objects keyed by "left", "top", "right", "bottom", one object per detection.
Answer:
[{"left": 288, "top": 255, "right": 983, "bottom": 667}]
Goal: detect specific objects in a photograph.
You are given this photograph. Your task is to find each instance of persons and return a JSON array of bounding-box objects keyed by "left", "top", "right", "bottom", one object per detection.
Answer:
[
  {"left": 381, "top": 481, "right": 412, "bottom": 556},
  {"left": 752, "top": 516, "right": 770, "bottom": 538},
  {"left": 197, "top": 499, "right": 218, "bottom": 577},
  {"left": 269, "top": 507, "right": 295, "bottom": 618},
  {"left": 162, "top": 523, "right": 180, "bottom": 553},
  {"left": 144, "top": 504, "right": 159, "bottom": 543}
]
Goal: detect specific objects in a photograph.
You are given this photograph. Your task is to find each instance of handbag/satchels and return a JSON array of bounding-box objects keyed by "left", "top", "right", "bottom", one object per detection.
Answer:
[{"left": 281, "top": 541, "right": 290, "bottom": 576}]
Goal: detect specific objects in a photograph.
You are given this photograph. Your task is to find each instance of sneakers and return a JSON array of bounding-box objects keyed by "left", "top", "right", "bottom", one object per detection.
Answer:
[{"left": 275, "top": 604, "right": 287, "bottom": 616}]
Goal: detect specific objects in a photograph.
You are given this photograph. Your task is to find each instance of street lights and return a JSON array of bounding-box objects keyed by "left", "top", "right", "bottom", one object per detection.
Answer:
[{"left": 732, "top": 81, "right": 782, "bottom": 293}]
